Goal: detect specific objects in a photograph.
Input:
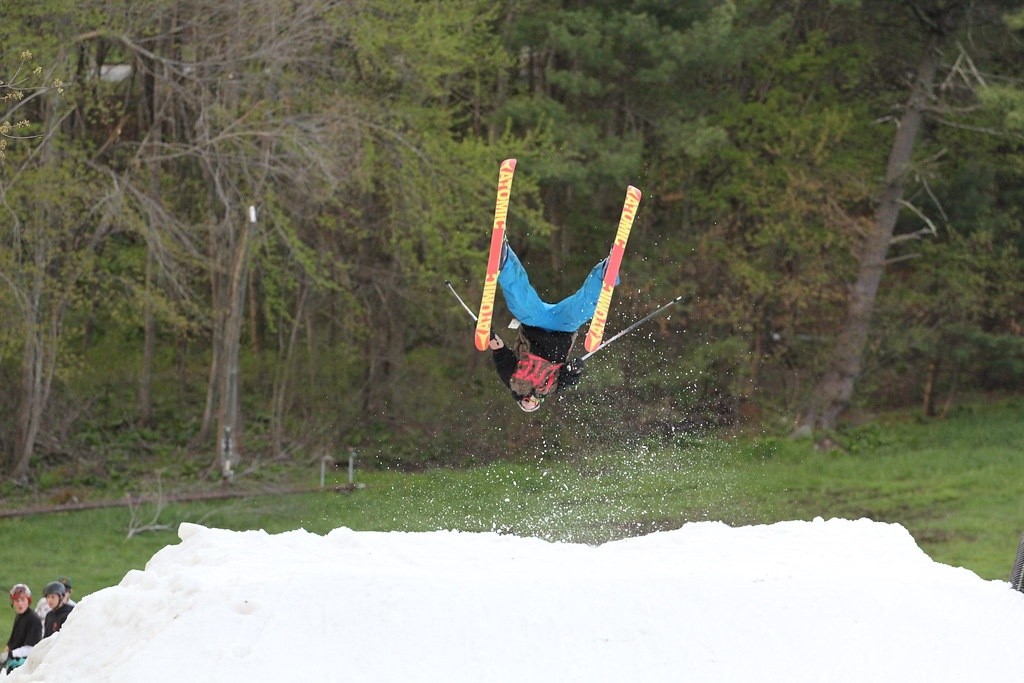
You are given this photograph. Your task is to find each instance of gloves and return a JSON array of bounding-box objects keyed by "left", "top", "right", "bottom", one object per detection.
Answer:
[{"left": 570, "top": 357, "right": 584, "bottom": 377}]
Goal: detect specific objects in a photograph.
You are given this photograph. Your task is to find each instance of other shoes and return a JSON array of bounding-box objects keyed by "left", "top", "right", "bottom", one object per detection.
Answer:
[
  {"left": 499, "top": 234, "right": 509, "bottom": 270},
  {"left": 603, "top": 243, "right": 615, "bottom": 280}
]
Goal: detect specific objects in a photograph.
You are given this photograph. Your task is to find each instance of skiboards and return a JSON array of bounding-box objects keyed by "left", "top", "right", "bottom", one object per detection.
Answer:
[{"left": 467, "top": 156, "right": 643, "bottom": 350}]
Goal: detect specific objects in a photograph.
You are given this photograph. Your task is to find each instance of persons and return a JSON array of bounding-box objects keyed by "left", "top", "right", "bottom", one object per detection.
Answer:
[
  {"left": 6, "top": 584, "right": 42, "bottom": 675},
  {"left": 489, "top": 234, "right": 621, "bottom": 412},
  {"left": 35, "top": 577, "right": 77, "bottom": 619},
  {"left": 43, "top": 582, "right": 74, "bottom": 638}
]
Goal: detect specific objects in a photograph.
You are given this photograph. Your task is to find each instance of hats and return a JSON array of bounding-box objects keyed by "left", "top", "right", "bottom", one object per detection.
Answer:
[{"left": 58, "top": 576, "right": 72, "bottom": 589}]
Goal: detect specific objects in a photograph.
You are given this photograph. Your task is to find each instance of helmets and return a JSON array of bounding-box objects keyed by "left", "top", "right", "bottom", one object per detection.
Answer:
[
  {"left": 517, "top": 401, "right": 541, "bottom": 413},
  {"left": 9, "top": 584, "right": 32, "bottom": 604},
  {"left": 43, "top": 582, "right": 65, "bottom": 597}
]
